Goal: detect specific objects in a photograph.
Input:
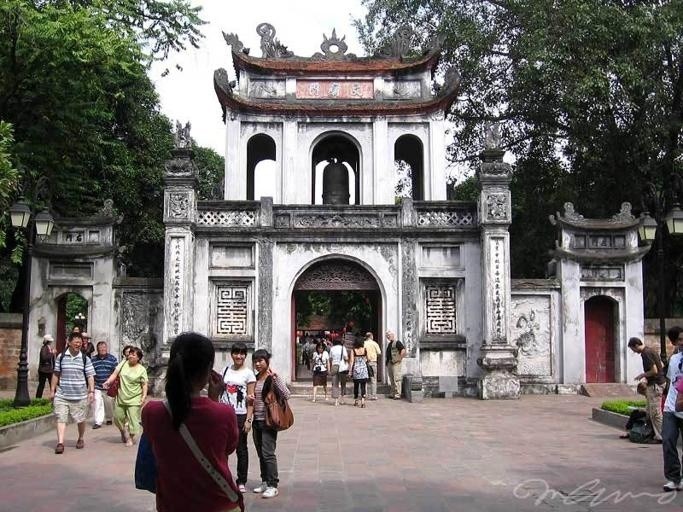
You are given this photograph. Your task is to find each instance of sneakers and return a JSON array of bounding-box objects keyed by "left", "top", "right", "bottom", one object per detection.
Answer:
[
  {"left": 122, "top": 434, "right": 136, "bottom": 447},
  {"left": 619, "top": 432, "right": 630, "bottom": 438},
  {"left": 239, "top": 484, "right": 245, "bottom": 493},
  {"left": 56, "top": 444, "right": 64, "bottom": 453},
  {"left": 664, "top": 481, "right": 683, "bottom": 491},
  {"left": 77, "top": 439, "right": 84, "bottom": 448},
  {"left": 107, "top": 421, "right": 112, "bottom": 425},
  {"left": 263, "top": 486, "right": 279, "bottom": 498},
  {"left": 254, "top": 482, "right": 267, "bottom": 493},
  {"left": 93, "top": 424, "right": 101, "bottom": 429}
]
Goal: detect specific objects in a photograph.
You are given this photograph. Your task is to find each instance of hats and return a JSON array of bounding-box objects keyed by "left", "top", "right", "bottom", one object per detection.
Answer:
[
  {"left": 43, "top": 334, "right": 55, "bottom": 342},
  {"left": 81, "top": 332, "right": 92, "bottom": 339}
]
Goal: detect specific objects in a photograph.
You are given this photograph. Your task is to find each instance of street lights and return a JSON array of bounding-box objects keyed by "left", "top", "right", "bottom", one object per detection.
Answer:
[
  {"left": 637, "top": 173, "right": 682, "bottom": 383},
  {"left": 75, "top": 312, "right": 86, "bottom": 330},
  {"left": 5, "top": 163, "right": 55, "bottom": 409}
]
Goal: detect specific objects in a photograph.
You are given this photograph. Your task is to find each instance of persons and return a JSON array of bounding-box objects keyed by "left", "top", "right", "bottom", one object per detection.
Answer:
[{"left": 628, "top": 326, "right": 683, "bottom": 491}]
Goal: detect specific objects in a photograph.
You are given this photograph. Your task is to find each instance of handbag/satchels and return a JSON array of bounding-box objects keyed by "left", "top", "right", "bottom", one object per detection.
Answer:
[
  {"left": 330, "top": 364, "right": 339, "bottom": 375},
  {"left": 107, "top": 377, "right": 120, "bottom": 397},
  {"left": 265, "top": 391, "right": 294, "bottom": 431},
  {"left": 631, "top": 417, "right": 653, "bottom": 444},
  {"left": 367, "top": 364, "right": 374, "bottom": 377},
  {"left": 312, "top": 363, "right": 327, "bottom": 372},
  {"left": 38, "top": 363, "right": 54, "bottom": 374}
]
